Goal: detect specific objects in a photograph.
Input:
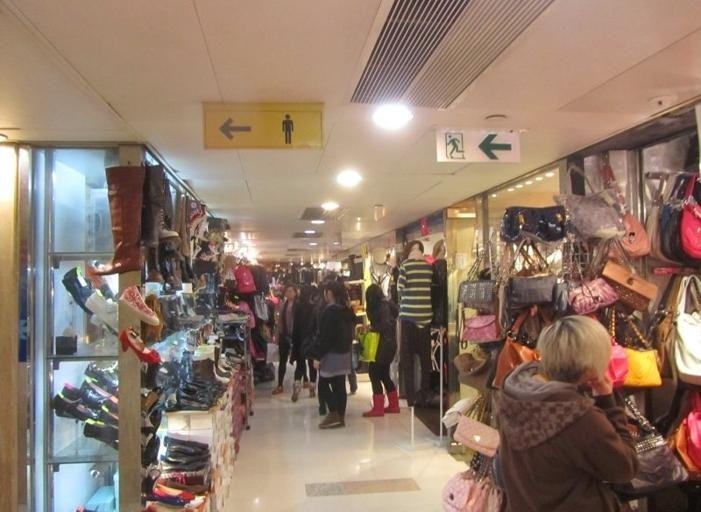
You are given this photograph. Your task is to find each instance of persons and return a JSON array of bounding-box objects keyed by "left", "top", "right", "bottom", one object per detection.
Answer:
[
  {"left": 271, "top": 280, "right": 357, "bottom": 431},
  {"left": 395, "top": 239, "right": 436, "bottom": 407},
  {"left": 361, "top": 284, "right": 401, "bottom": 417},
  {"left": 493, "top": 316, "right": 638, "bottom": 512},
  {"left": 432, "top": 240, "right": 446, "bottom": 329},
  {"left": 446, "top": 134, "right": 464, "bottom": 159}
]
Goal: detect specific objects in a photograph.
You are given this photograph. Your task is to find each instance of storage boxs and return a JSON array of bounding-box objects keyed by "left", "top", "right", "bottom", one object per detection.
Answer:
[{"left": 168, "top": 309, "right": 254, "bottom": 508}]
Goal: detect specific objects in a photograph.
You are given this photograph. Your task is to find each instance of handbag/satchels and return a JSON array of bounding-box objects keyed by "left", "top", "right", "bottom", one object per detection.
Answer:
[
  {"left": 356, "top": 332, "right": 381, "bottom": 362},
  {"left": 239, "top": 263, "right": 275, "bottom": 383},
  {"left": 441, "top": 163, "right": 700, "bottom": 509}
]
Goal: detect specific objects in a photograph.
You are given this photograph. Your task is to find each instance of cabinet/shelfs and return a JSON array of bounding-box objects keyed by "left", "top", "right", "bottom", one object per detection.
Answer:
[
  {"left": 30, "top": 142, "right": 217, "bottom": 512},
  {"left": 347, "top": 279, "right": 367, "bottom": 345}
]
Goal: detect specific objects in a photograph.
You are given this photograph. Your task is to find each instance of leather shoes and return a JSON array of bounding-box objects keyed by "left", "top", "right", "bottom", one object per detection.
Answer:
[{"left": 52, "top": 261, "right": 243, "bottom": 512}]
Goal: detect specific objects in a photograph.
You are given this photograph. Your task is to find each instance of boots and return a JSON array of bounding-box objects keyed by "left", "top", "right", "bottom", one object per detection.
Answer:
[
  {"left": 91, "top": 163, "right": 194, "bottom": 294},
  {"left": 364, "top": 395, "right": 384, "bottom": 416},
  {"left": 384, "top": 390, "right": 399, "bottom": 413}
]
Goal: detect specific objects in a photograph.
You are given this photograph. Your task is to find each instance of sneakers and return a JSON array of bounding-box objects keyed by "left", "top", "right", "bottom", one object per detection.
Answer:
[
  {"left": 271, "top": 381, "right": 315, "bottom": 401},
  {"left": 320, "top": 412, "right": 341, "bottom": 428}
]
